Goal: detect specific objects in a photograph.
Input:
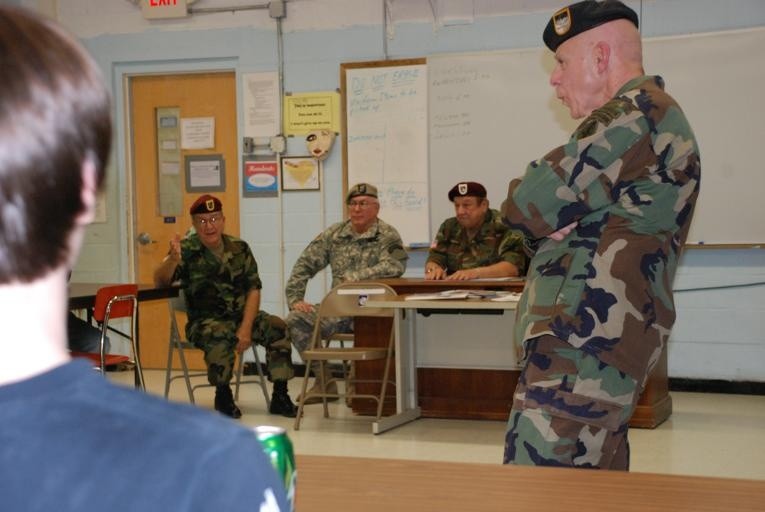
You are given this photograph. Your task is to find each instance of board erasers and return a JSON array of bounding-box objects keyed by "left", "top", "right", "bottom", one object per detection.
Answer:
[{"left": 409, "top": 243, "right": 430, "bottom": 248}]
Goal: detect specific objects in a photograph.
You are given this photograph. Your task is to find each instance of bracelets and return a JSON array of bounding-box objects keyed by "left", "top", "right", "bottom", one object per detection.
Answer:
[{"left": 425, "top": 265, "right": 442, "bottom": 273}]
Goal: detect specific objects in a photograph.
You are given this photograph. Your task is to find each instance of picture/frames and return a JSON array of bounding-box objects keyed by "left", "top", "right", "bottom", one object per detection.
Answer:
[{"left": 279, "top": 156, "right": 322, "bottom": 192}]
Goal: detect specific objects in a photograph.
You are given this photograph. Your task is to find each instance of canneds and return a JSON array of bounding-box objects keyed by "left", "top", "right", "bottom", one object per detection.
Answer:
[{"left": 254, "top": 425, "right": 297, "bottom": 512}]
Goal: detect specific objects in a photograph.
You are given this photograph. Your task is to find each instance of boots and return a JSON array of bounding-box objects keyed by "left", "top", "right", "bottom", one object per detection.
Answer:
[
  {"left": 268, "top": 379, "right": 304, "bottom": 419},
  {"left": 295, "top": 363, "right": 340, "bottom": 404},
  {"left": 345, "top": 366, "right": 356, "bottom": 407},
  {"left": 214, "top": 384, "right": 243, "bottom": 418}
]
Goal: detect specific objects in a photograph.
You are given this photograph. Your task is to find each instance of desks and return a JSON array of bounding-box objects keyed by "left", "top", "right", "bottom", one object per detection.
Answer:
[
  {"left": 290, "top": 447, "right": 764, "bottom": 512},
  {"left": 67, "top": 278, "right": 180, "bottom": 391},
  {"left": 352, "top": 267, "right": 674, "bottom": 436}
]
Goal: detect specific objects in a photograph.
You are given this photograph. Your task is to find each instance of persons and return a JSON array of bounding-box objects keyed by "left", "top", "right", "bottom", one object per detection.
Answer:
[
  {"left": 152, "top": 195, "right": 304, "bottom": 420},
  {"left": 285, "top": 183, "right": 410, "bottom": 405},
  {"left": 496, "top": 0, "right": 701, "bottom": 469},
  {"left": 0, "top": 1, "right": 288, "bottom": 511},
  {"left": 424, "top": 182, "right": 528, "bottom": 281}
]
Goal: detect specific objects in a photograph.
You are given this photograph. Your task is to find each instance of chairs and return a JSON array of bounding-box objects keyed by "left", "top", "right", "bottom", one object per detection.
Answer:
[
  {"left": 161, "top": 270, "right": 274, "bottom": 415},
  {"left": 67, "top": 280, "right": 146, "bottom": 394},
  {"left": 290, "top": 277, "right": 403, "bottom": 432}
]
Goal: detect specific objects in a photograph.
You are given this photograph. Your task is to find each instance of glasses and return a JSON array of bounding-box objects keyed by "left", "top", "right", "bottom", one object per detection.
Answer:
[
  {"left": 348, "top": 199, "right": 375, "bottom": 208},
  {"left": 195, "top": 216, "right": 221, "bottom": 224}
]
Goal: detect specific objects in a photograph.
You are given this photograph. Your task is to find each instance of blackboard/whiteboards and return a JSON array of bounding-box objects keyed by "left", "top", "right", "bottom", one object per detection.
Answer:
[
  {"left": 339, "top": 57, "right": 431, "bottom": 252},
  {"left": 425, "top": 26, "right": 765, "bottom": 253}
]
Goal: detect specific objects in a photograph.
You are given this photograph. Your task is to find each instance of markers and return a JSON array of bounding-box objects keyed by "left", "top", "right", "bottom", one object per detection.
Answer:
[{"left": 685, "top": 242, "right": 704, "bottom": 245}]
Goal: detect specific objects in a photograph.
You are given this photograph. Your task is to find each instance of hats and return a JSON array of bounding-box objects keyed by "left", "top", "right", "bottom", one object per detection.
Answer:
[
  {"left": 447, "top": 182, "right": 487, "bottom": 201},
  {"left": 542, "top": 0, "right": 639, "bottom": 53},
  {"left": 189, "top": 194, "right": 223, "bottom": 215},
  {"left": 345, "top": 182, "right": 378, "bottom": 204}
]
